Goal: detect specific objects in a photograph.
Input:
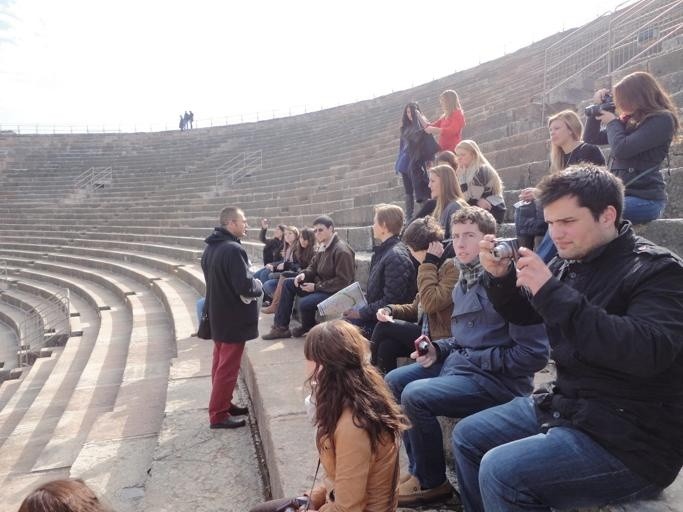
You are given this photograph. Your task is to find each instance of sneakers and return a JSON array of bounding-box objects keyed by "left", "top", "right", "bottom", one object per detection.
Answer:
[
  {"left": 262, "top": 323, "right": 292, "bottom": 339},
  {"left": 398, "top": 475, "right": 453, "bottom": 506},
  {"left": 293, "top": 326, "right": 307, "bottom": 336}
]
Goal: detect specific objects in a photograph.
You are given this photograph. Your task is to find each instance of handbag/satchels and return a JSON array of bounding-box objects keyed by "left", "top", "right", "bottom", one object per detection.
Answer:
[
  {"left": 407, "top": 128, "right": 440, "bottom": 160},
  {"left": 198, "top": 307, "right": 211, "bottom": 339}
]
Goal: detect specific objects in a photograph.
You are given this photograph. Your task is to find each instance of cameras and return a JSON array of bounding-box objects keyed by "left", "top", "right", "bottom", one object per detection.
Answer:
[
  {"left": 271, "top": 499, "right": 299, "bottom": 512},
  {"left": 415, "top": 335, "right": 429, "bottom": 356},
  {"left": 585, "top": 94, "right": 616, "bottom": 116},
  {"left": 490, "top": 237, "right": 521, "bottom": 261}
]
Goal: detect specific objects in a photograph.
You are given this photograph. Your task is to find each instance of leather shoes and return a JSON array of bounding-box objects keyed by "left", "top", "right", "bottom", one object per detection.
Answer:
[
  {"left": 211, "top": 416, "right": 245, "bottom": 428},
  {"left": 229, "top": 406, "right": 248, "bottom": 415}
]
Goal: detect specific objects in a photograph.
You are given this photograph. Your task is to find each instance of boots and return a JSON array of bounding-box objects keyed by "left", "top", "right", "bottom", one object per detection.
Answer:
[
  {"left": 261, "top": 274, "right": 285, "bottom": 313},
  {"left": 404, "top": 194, "right": 414, "bottom": 225}
]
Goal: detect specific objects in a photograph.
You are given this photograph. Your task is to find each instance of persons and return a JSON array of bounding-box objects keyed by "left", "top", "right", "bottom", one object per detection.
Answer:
[
  {"left": 178, "top": 115, "right": 184, "bottom": 131},
  {"left": 535, "top": 71, "right": 679, "bottom": 263},
  {"left": 183, "top": 111, "right": 189, "bottom": 131},
  {"left": 515, "top": 110, "right": 606, "bottom": 251},
  {"left": 188, "top": 111, "right": 193, "bottom": 129},
  {"left": 249, "top": 139, "right": 682, "bottom": 511},
  {"left": 416, "top": 90, "right": 466, "bottom": 152},
  {"left": 18, "top": 479, "right": 114, "bottom": 511},
  {"left": 201, "top": 206, "right": 263, "bottom": 428},
  {"left": 395, "top": 103, "right": 435, "bottom": 223}
]
